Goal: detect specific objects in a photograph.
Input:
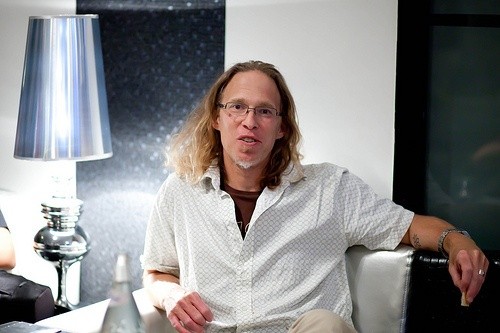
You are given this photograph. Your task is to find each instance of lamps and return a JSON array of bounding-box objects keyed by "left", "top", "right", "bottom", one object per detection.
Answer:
[{"left": 14, "top": 14, "right": 114, "bottom": 309}]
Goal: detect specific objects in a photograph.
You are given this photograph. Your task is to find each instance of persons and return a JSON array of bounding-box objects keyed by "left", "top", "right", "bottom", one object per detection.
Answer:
[
  {"left": 0, "top": 210, "right": 56, "bottom": 319},
  {"left": 142, "top": 61, "right": 489, "bottom": 333}
]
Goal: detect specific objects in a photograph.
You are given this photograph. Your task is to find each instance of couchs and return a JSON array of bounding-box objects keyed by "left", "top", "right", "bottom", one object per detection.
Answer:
[{"left": 34, "top": 242, "right": 500, "bottom": 333}]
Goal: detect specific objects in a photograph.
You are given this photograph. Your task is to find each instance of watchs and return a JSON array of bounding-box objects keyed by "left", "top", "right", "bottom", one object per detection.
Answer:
[{"left": 438, "top": 230, "right": 470, "bottom": 259}]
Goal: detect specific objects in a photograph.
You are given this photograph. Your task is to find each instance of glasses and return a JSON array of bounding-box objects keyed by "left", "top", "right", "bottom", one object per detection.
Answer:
[{"left": 216, "top": 102, "right": 283, "bottom": 120}]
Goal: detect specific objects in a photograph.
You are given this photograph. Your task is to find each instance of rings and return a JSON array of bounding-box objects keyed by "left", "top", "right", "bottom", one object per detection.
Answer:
[{"left": 479, "top": 268, "right": 485, "bottom": 276}]
